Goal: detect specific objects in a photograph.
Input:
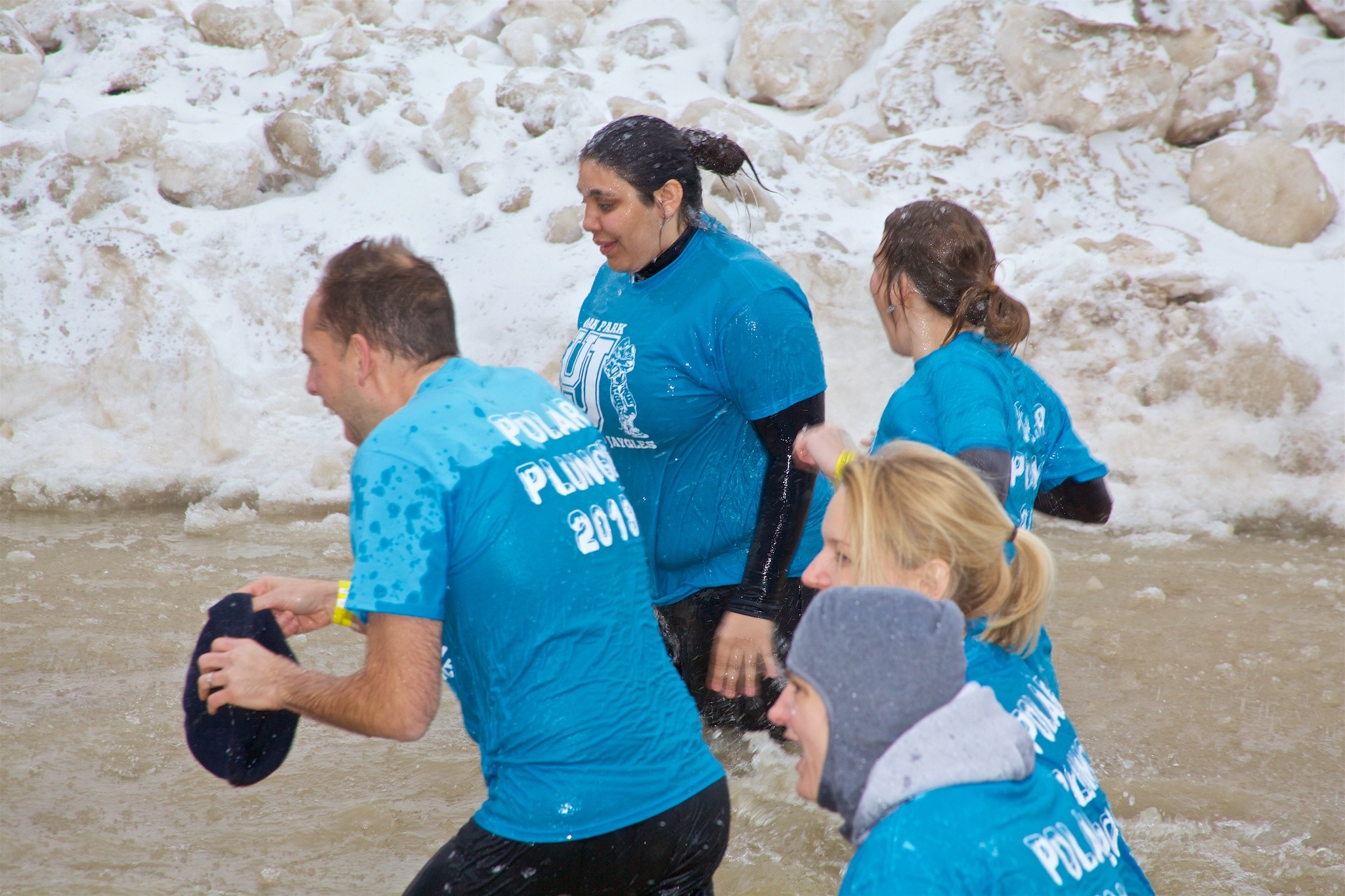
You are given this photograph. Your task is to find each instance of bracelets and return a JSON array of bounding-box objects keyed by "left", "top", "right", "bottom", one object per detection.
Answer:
[
  {"left": 836, "top": 450, "right": 856, "bottom": 480},
  {"left": 332, "top": 580, "right": 353, "bottom": 626}
]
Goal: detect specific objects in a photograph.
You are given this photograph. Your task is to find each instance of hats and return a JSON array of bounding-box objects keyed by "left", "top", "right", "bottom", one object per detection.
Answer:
[{"left": 184, "top": 592, "right": 301, "bottom": 787}]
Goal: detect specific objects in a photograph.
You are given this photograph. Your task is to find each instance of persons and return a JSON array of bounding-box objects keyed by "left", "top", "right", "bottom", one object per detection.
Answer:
[
  {"left": 557, "top": 115, "right": 826, "bottom": 742},
  {"left": 767, "top": 586, "right": 1126, "bottom": 896},
  {"left": 801, "top": 439, "right": 1153, "bottom": 896},
  {"left": 198, "top": 243, "right": 731, "bottom": 896},
  {"left": 795, "top": 201, "right": 1113, "bottom": 696}
]
